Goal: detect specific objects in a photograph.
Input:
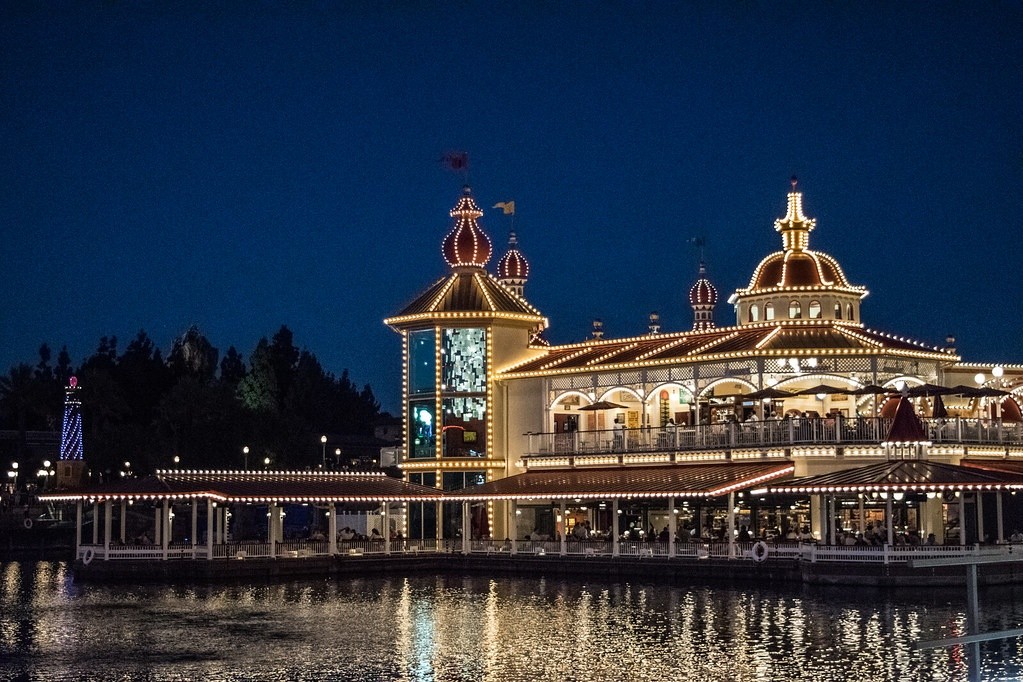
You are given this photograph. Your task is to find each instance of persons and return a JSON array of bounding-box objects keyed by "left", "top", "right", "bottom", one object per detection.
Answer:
[
  {"left": 113, "top": 527, "right": 404, "bottom": 555},
  {"left": 619, "top": 523, "right": 668, "bottom": 543},
  {"left": 892, "top": 528, "right": 938, "bottom": 547},
  {"left": 676, "top": 518, "right": 727, "bottom": 542},
  {"left": 515, "top": 519, "right": 612, "bottom": 541},
  {"left": 736, "top": 516, "right": 812, "bottom": 542},
  {"left": 598, "top": 409, "right": 1017, "bottom": 446},
  {"left": 451, "top": 528, "right": 511, "bottom": 551},
  {"left": 942, "top": 524, "right": 1023, "bottom": 546},
  {"left": 835, "top": 520, "right": 887, "bottom": 547}
]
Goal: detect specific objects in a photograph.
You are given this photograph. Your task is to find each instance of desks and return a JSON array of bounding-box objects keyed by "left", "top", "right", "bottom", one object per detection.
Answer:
[
  {"left": 680, "top": 428, "right": 696, "bottom": 451},
  {"left": 655, "top": 432, "right": 667, "bottom": 450}
]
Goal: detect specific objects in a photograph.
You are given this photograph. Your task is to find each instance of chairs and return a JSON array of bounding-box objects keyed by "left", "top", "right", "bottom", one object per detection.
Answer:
[{"left": 549, "top": 409, "right": 873, "bottom": 457}]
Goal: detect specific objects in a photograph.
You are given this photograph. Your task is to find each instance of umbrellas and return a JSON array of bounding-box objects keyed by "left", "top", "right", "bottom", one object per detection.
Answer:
[{"left": 575, "top": 383, "right": 1016, "bottom": 433}]
